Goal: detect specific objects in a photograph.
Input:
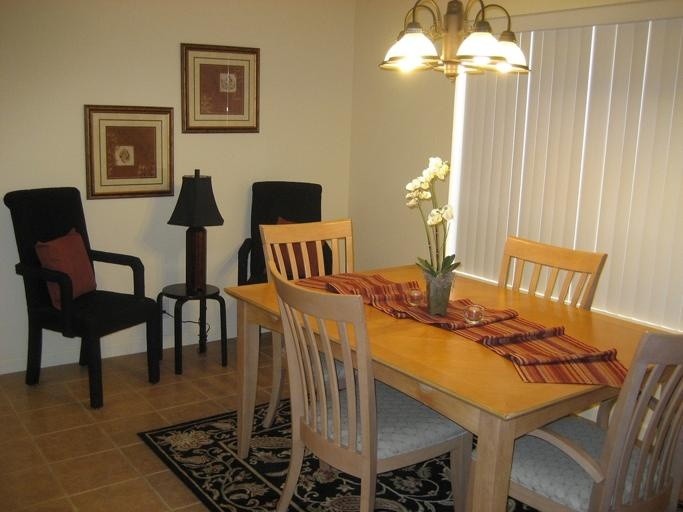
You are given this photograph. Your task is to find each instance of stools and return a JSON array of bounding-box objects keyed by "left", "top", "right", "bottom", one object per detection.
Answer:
[{"left": 157, "top": 283, "right": 228, "bottom": 375}]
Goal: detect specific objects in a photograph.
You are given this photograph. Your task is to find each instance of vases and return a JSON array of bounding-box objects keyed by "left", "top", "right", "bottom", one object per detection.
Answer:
[{"left": 426, "top": 272, "right": 453, "bottom": 315}]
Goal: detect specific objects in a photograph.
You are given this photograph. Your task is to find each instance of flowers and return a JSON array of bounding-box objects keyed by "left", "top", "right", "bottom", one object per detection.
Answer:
[{"left": 403, "top": 157, "right": 461, "bottom": 277}]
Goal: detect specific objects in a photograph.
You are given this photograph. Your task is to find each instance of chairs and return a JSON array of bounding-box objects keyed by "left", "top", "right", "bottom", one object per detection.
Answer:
[
  {"left": 497, "top": 236, "right": 608, "bottom": 312},
  {"left": 258, "top": 219, "right": 369, "bottom": 430},
  {"left": 3, "top": 188, "right": 159, "bottom": 409},
  {"left": 269, "top": 262, "right": 472, "bottom": 512},
  {"left": 466, "top": 331, "right": 683, "bottom": 512},
  {"left": 236, "top": 181, "right": 333, "bottom": 286}
]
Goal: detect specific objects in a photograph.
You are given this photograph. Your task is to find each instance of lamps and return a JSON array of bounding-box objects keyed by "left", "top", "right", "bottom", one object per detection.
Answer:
[
  {"left": 166, "top": 169, "right": 223, "bottom": 293},
  {"left": 378, "top": 0, "right": 531, "bottom": 271}
]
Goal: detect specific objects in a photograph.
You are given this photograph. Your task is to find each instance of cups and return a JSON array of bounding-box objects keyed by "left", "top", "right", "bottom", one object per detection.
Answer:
[
  {"left": 406, "top": 287, "right": 424, "bottom": 307},
  {"left": 463, "top": 304, "right": 483, "bottom": 324}
]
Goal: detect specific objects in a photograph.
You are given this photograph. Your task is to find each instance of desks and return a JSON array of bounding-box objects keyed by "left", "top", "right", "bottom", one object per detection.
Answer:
[{"left": 224, "top": 259, "right": 683, "bottom": 512}]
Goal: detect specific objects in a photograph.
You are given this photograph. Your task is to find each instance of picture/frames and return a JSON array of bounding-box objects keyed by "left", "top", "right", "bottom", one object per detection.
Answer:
[
  {"left": 181, "top": 43, "right": 260, "bottom": 134},
  {"left": 83, "top": 105, "right": 174, "bottom": 200}
]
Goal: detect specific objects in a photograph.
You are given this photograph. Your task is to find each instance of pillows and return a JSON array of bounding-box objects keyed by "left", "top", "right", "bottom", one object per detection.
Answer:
[
  {"left": 34, "top": 227, "right": 96, "bottom": 311},
  {"left": 271, "top": 217, "right": 327, "bottom": 276}
]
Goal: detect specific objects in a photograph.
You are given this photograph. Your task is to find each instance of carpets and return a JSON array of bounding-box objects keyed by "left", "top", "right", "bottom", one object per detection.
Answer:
[{"left": 137, "top": 378, "right": 540, "bottom": 511}]
